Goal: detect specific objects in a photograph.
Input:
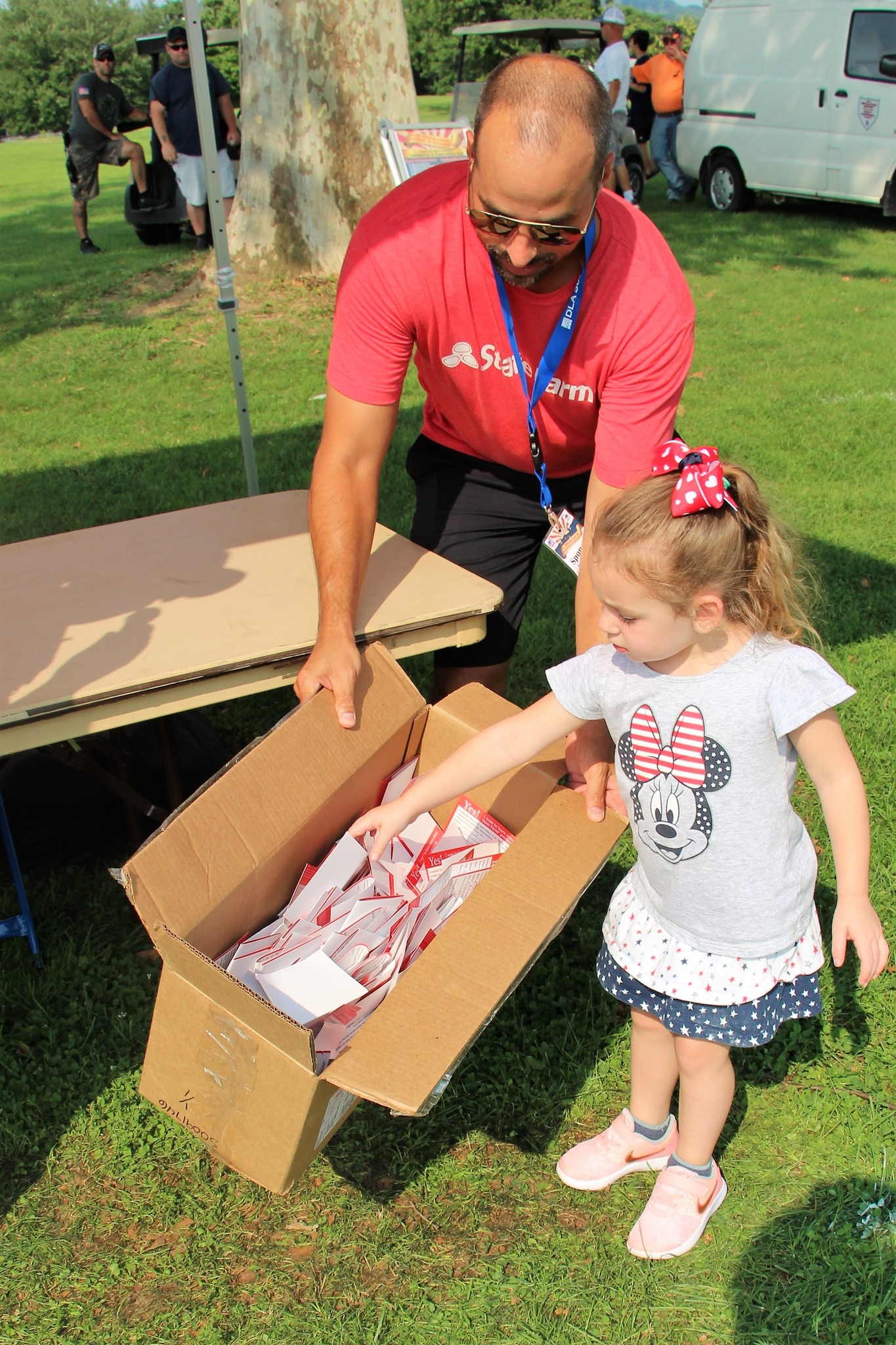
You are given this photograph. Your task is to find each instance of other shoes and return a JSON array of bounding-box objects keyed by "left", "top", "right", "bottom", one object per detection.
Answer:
[
  {"left": 666, "top": 189, "right": 680, "bottom": 200},
  {"left": 193, "top": 237, "right": 210, "bottom": 250},
  {"left": 632, "top": 199, "right": 641, "bottom": 210},
  {"left": 139, "top": 195, "right": 169, "bottom": 211},
  {"left": 628, "top": 1153, "right": 728, "bottom": 1257},
  {"left": 555, "top": 1107, "right": 678, "bottom": 1190},
  {"left": 80, "top": 239, "right": 100, "bottom": 253}
]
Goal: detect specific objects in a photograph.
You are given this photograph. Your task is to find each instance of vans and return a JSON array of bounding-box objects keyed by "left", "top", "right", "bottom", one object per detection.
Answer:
[{"left": 670, "top": 1, "right": 895, "bottom": 223}]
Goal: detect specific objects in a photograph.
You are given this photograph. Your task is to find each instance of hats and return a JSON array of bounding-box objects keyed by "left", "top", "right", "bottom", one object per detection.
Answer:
[
  {"left": 167, "top": 26, "right": 188, "bottom": 43},
  {"left": 92, "top": 43, "right": 114, "bottom": 59},
  {"left": 661, "top": 25, "right": 682, "bottom": 41},
  {"left": 592, "top": 7, "right": 626, "bottom": 24}
]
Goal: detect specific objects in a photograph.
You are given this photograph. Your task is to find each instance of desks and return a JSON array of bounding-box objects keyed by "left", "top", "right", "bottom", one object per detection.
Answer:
[{"left": 0, "top": 489, "right": 505, "bottom": 954}]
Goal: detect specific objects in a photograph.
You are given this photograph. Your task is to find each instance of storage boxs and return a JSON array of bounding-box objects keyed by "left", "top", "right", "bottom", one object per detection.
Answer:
[{"left": 107, "top": 640, "right": 631, "bottom": 1196}]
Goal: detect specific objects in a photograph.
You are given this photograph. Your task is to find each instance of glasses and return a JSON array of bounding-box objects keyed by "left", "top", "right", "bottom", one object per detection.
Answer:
[
  {"left": 167, "top": 42, "right": 188, "bottom": 51},
  {"left": 464, "top": 142, "right": 602, "bottom": 247},
  {"left": 97, "top": 55, "right": 115, "bottom": 62},
  {"left": 600, "top": 21, "right": 614, "bottom": 27},
  {"left": 664, "top": 36, "right": 678, "bottom": 45}
]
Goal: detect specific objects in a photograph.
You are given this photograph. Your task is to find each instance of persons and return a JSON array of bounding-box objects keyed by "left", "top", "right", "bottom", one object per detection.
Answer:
[
  {"left": 630, "top": 24, "right": 694, "bottom": 203},
  {"left": 149, "top": 26, "right": 243, "bottom": 249},
  {"left": 63, "top": 43, "right": 149, "bottom": 253},
  {"left": 566, "top": 55, "right": 581, "bottom": 64},
  {"left": 627, "top": 29, "right": 655, "bottom": 179},
  {"left": 296, "top": 52, "right": 697, "bottom": 825},
  {"left": 592, "top": 7, "right": 639, "bottom": 210},
  {"left": 353, "top": 435, "right": 888, "bottom": 1263}
]
